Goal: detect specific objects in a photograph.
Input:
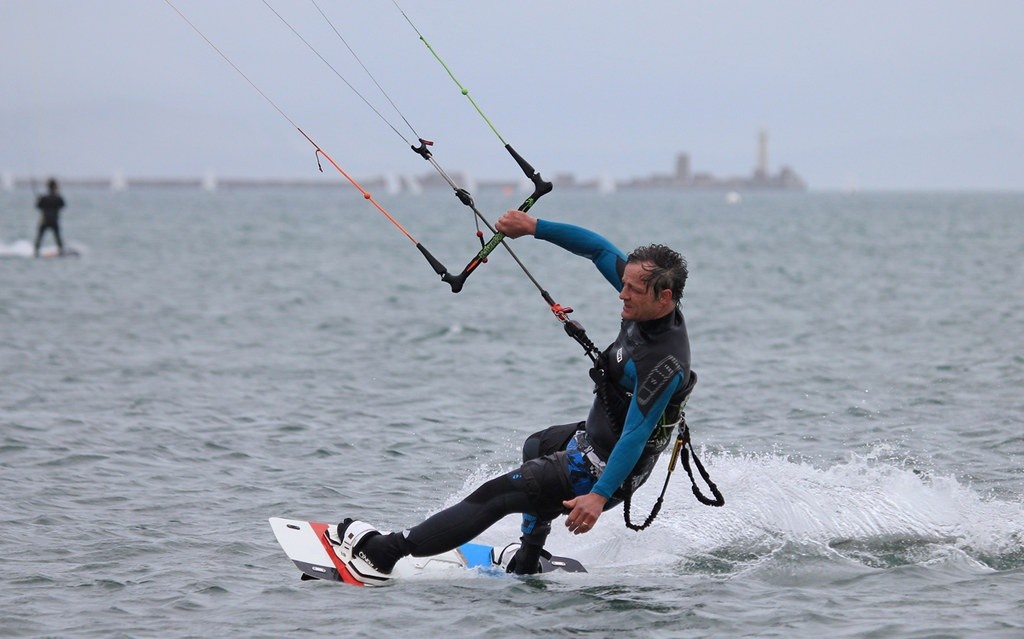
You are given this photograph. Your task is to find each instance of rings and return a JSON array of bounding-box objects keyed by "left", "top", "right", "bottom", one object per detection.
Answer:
[{"left": 583, "top": 522, "right": 588, "bottom": 525}]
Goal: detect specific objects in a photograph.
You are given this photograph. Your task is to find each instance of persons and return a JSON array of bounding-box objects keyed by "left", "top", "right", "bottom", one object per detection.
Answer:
[
  {"left": 338, "top": 209, "right": 692, "bottom": 575},
  {"left": 34, "top": 178, "right": 64, "bottom": 256}
]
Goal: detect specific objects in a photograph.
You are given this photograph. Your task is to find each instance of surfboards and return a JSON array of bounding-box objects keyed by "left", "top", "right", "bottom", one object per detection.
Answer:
[
  {"left": 267, "top": 515, "right": 589, "bottom": 588},
  {"left": 45, "top": 248, "right": 82, "bottom": 258}
]
